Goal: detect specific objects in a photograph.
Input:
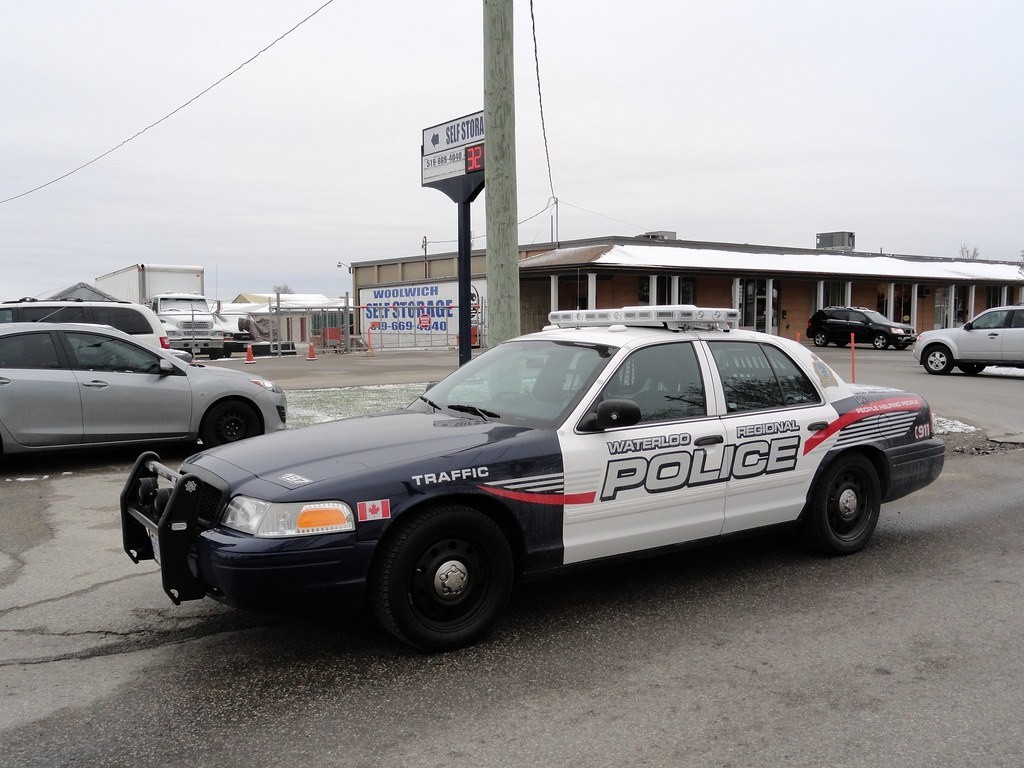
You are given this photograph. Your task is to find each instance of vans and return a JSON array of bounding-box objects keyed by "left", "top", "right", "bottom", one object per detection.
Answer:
[{"left": 1, "top": 297, "right": 171, "bottom": 350}]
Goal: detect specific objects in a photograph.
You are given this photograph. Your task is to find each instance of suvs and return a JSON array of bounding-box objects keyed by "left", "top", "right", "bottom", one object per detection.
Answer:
[
  {"left": 913, "top": 306, "right": 1024, "bottom": 376},
  {"left": 806, "top": 304, "right": 917, "bottom": 351}
]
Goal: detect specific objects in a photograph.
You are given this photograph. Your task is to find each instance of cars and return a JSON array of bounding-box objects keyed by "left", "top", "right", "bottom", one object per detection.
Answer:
[
  {"left": 119, "top": 306, "right": 950, "bottom": 652},
  {"left": 1, "top": 319, "right": 289, "bottom": 467}
]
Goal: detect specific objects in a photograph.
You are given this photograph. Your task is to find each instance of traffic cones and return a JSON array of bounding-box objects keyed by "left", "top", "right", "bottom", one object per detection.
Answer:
[
  {"left": 306, "top": 339, "right": 317, "bottom": 361},
  {"left": 244, "top": 342, "right": 256, "bottom": 364}
]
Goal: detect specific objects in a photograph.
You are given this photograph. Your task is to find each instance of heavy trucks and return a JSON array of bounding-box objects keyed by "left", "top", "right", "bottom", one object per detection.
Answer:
[{"left": 93, "top": 264, "right": 224, "bottom": 359}]
